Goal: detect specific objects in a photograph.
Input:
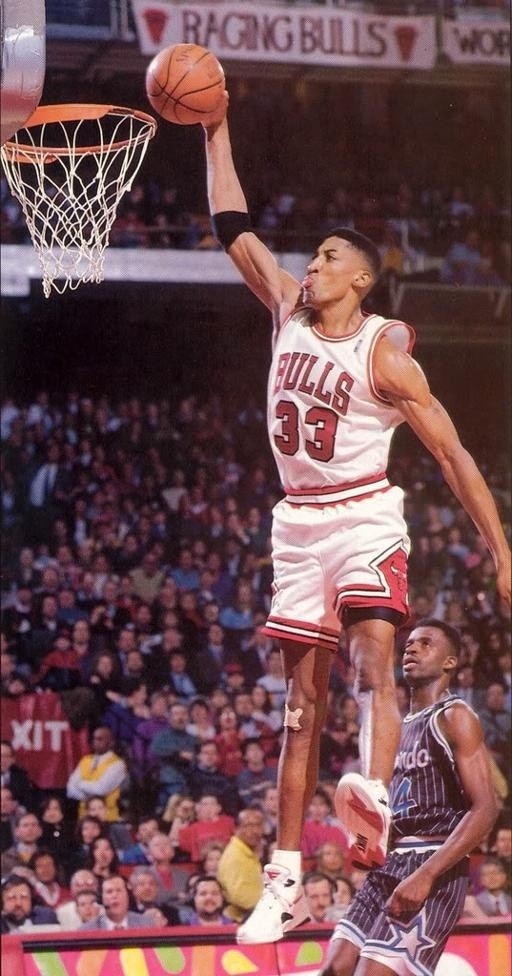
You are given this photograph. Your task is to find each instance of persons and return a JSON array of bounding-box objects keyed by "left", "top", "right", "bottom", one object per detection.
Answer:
[
  {"left": 1, "top": 384, "right": 510, "bottom": 976},
  {"left": 201, "top": 93, "right": 511, "bottom": 944},
  {"left": 1, "top": 54, "right": 511, "bottom": 285}
]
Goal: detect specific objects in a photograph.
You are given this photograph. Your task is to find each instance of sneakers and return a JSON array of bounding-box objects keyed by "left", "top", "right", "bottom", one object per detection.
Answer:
[
  {"left": 235, "top": 862, "right": 311, "bottom": 946},
  {"left": 333, "top": 773, "right": 392, "bottom": 871}
]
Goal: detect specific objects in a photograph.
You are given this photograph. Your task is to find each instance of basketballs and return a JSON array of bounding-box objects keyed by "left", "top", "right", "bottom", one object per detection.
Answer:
[{"left": 146, "top": 43, "right": 224, "bottom": 124}]
